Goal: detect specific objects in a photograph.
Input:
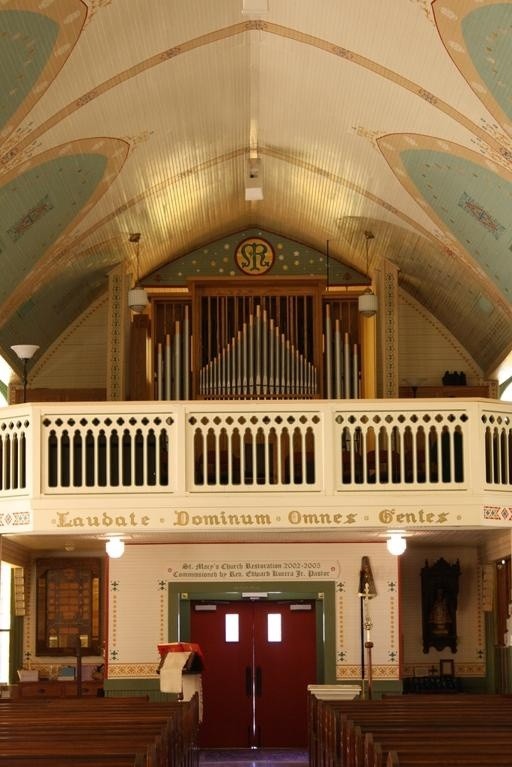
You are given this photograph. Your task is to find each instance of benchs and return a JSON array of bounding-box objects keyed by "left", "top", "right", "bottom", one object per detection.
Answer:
[
  {"left": 0, "top": 695, "right": 200, "bottom": 766},
  {"left": 307, "top": 685, "right": 511, "bottom": 766}
]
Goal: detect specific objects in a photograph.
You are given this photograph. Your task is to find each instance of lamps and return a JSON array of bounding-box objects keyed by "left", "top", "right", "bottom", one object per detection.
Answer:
[
  {"left": 11, "top": 343, "right": 41, "bottom": 402},
  {"left": 385, "top": 532, "right": 409, "bottom": 557},
  {"left": 357, "top": 231, "right": 379, "bottom": 318},
  {"left": 106, "top": 538, "right": 124, "bottom": 559},
  {"left": 64, "top": 540, "right": 77, "bottom": 552},
  {"left": 126, "top": 233, "right": 149, "bottom": 314}
]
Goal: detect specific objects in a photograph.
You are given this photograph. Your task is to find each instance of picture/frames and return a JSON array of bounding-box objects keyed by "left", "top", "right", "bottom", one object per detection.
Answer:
[{"left": 414, "top": 659, "right": 454, "bottom": 678}]
